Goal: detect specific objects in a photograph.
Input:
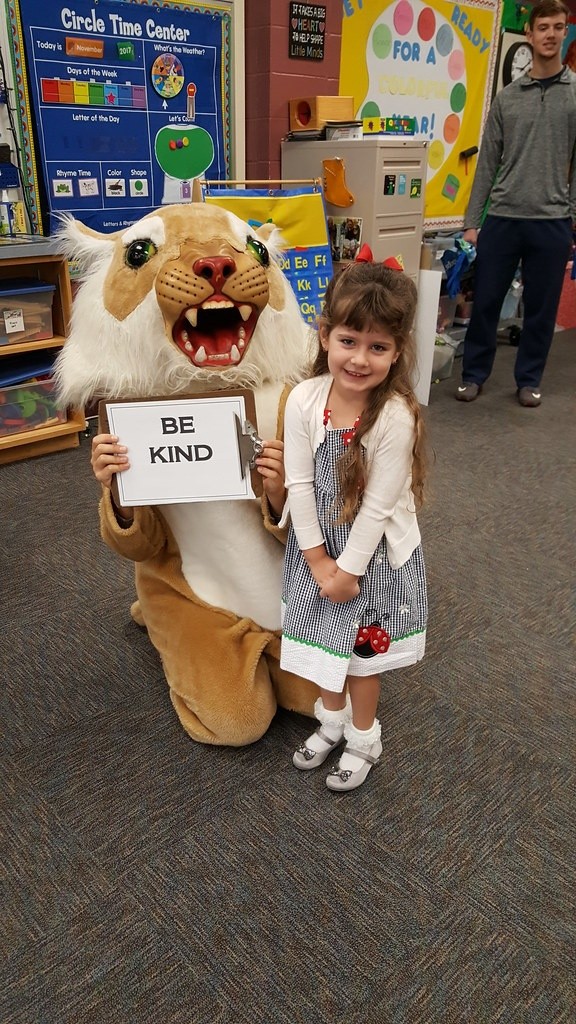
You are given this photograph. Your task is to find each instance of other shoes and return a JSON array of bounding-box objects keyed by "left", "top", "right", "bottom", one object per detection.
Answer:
[
  {"left": 326, "top": 740, "right": 383, "bottom": 791},
  {"left": 455, "top": 382, "right": 480, "bottom": 401},
  {"left": 518, "top": 386, "right": 543, "bottom": 408}
]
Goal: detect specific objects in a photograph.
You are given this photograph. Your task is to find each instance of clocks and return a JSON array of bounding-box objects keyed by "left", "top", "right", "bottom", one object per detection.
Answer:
[{"left": 503, "top": 42, "right": 534, "bottom": 85}]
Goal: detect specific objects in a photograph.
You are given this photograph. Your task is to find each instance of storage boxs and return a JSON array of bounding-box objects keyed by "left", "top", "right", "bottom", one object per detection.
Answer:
[
  {"left": 0, "top": 285, "right": 55, "bottom": 345},
  {"left": 0, "top": 366, "right": 67, "bottom": 436},
  {"left": 437, "top": 296, "right": 455, "bottom": 329},
  {"left": 364, "top": 117, "right": 415, "bottom": 135},
  {"left": 431, "top": 334, "right": 459, "bottom": 382},
  {"left": 554, "top": 260, "right": 576, "bottom": 333},
  {"left": 0, "top": 202, "right": 27, "bottom": 236}
]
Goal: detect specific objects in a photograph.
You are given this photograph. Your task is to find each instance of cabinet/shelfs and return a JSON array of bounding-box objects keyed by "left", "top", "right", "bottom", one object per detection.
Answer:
[{"left": 0, "top": 256, "right": 86, "bottom": 464}]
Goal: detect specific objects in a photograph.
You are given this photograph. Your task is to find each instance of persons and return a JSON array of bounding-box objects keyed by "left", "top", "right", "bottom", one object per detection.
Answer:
[
  {"left": 50, "top": 200, "right": 322, "bottom": 745},
  {"left": 453, "top": 1, "right": 576, "bottom": 407},
  {"left": 280, "top": 243, "right": 428, "bottom": 794}
]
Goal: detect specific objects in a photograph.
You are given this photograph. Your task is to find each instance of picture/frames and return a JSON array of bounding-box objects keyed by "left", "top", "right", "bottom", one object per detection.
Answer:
[{"left": 491, "top": 27, "right": 529, "bottom": 102}]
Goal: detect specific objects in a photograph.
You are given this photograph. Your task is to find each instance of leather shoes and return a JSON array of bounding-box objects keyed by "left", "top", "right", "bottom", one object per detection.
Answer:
[{"left": 293, "top": 726, "right": 345, "bottom": 769}]
